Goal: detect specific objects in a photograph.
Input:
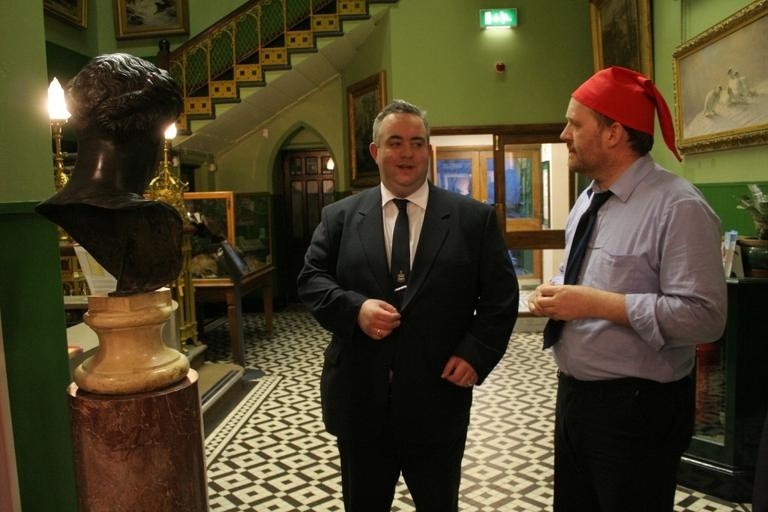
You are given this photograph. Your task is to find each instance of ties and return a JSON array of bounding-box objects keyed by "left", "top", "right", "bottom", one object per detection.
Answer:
[
  {"left": 544, "top": 190, "right": 614, "bottom": 350},
  {"left": 391, "top": 199, "right": 410, "bottom": 302}
]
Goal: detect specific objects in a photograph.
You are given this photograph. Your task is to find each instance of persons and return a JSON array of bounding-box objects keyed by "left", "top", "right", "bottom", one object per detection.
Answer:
[
  {"left": 523, "top": 65, "right": 728, "bottom": 512},
  {"left": 294, "top": 98, "right": 520, "bottom": 512}
]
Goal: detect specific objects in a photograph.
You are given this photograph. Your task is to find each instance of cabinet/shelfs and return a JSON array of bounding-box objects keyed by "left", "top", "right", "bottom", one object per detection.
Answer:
[{"left": 675, "top": 277, "right": 767, "bottom": 504}]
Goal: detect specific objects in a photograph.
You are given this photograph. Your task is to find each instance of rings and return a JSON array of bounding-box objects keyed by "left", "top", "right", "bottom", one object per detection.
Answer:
[
  {"left": 468, "top": 380, "right": 473, "bottom": 387},
  {"left": 376, "top": 329, "right": 382, "bottom": 337}
]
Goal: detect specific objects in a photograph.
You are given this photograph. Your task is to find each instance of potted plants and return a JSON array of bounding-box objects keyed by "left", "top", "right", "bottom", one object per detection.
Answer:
[{"left": 735, "top": 184, "right": 768, "bottom": 278}]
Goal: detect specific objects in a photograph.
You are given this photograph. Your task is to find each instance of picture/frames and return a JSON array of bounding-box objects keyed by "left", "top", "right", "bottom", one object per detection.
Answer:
[
  {"left": 672, "top": 2, "right": 768, "bottom": 156},
  {"left": 42, "top": 0, "right": 90, "bottom": 29},
  {"left": 587, "top": 0, "right": 655, "bottom": 85},
  {"left": 344, "top": 68, "right": 387, "bottom": 188},
  {"left": 539, "top": 160, "right": 551, "bottom": 228},
  {"left": 112, "top": 0, "right": 191, "bottom": 41}
]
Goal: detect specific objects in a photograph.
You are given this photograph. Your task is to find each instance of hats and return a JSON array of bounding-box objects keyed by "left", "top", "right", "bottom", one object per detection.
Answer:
[{"left": 571, "top": 66, "right": 682, "bottom": 161}]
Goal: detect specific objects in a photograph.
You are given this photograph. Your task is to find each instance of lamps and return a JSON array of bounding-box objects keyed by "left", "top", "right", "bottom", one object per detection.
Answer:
[
  {"left": 147, "top": 118, "right": 192, "bottom": 227},
  {"left": 46, "top": 74, "right": 72, "bottom": 242}
]
